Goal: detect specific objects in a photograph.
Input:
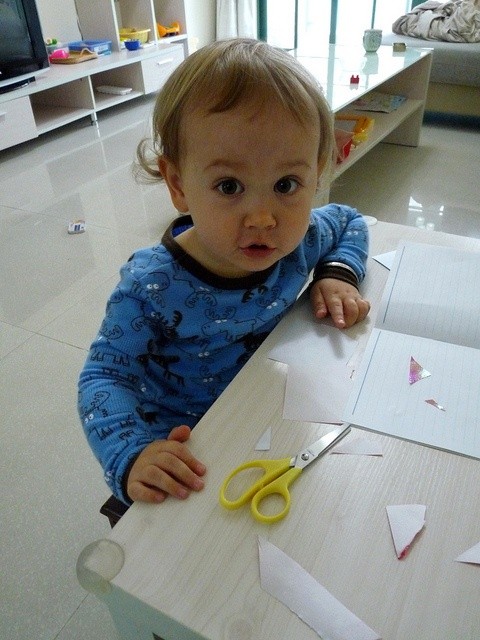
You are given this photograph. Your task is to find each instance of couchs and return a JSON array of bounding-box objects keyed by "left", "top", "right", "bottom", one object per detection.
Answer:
[{"left": 378, "top": 32, "right": 480, "bottom": 123}]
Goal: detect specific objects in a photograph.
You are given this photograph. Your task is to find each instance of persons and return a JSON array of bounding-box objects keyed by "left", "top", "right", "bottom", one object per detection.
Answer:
[{"left": 78, "top": 37, "right": 371, "bottom": 509}]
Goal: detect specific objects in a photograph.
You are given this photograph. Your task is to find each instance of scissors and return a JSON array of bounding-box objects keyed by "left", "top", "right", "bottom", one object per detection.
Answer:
[{"left": 222, "top": 422, "right": 353, "bottom": 523}]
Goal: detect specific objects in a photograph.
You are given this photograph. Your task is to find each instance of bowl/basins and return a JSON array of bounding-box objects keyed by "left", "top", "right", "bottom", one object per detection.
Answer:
[{"left": 123, "top": 38, "right": 141, "bottom": 51}]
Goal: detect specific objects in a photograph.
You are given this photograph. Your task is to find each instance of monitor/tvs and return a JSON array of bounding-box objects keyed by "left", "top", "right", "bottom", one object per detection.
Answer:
[{"left": 0, "top": 1, "right": 51, "bottom": 96}]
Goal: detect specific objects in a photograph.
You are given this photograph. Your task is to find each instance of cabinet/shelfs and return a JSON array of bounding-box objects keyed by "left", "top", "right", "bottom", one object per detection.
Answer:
[
  {"left": 75, "top": 0, "right": 190, "bottom": 59},
  {"left": 0, "top": 43, "right": 185, "bottom": 153}
]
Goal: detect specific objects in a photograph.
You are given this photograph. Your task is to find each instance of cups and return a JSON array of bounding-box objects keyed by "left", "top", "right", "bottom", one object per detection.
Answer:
[{"left": 362, "top": 28, "right": 383, "bottom": 52}]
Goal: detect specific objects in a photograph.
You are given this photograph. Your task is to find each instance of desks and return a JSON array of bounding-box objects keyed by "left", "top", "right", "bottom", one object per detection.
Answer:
[{"left": 73, "top": 214, "right": 479, "bottom": 639}]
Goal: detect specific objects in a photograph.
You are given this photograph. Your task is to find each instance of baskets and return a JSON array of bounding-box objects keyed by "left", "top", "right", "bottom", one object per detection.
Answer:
[{"left": 119, "top": 28, "right": 151, "bottom": 44}]
